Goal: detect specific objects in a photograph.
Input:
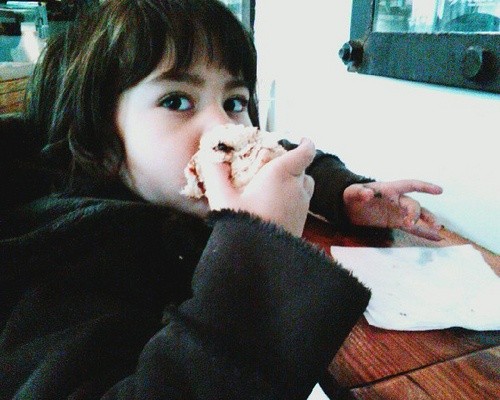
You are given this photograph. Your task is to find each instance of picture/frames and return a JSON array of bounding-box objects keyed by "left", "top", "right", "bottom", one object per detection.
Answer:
[{"left": 336, "top": 0, "right": 499, "bottom": 95}]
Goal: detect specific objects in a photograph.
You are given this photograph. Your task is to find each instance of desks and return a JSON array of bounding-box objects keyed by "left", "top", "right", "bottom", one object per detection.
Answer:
[{"left": 296, "top": 205, "right": 499, "bottom": 400}]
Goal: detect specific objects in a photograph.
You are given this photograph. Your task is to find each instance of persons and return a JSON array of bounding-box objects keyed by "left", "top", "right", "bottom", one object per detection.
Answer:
[{"left": 2, "top": 0, "right": 443, "bottom": 399}]
